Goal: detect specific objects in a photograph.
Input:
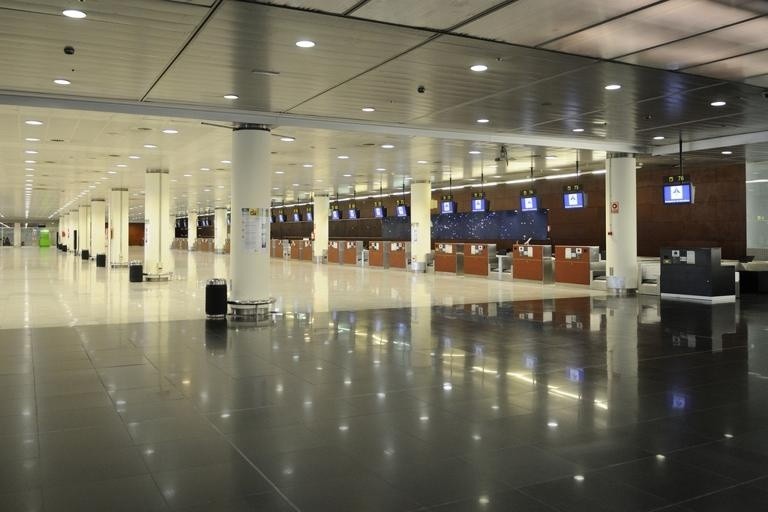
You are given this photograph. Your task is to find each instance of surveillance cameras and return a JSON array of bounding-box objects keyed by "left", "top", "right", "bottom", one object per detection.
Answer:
[
  {"left": 64, "top": 47, "right": 74, "bottom": 55},
  {"left": 418, "top": 87, "right": 425, "bottom": 93}
]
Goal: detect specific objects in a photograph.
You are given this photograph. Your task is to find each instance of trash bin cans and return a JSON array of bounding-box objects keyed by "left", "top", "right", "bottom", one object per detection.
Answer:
[
  {"left": 129, "top": 261, "right": 143, "bottom": 282},
  {"left": 59, "top": 244, "right": 67, "bottom": 251},
  {"left": 205, "top": 278, "right": 227, "bottom": 320},
  {"left": 97, "top": 254, "right": 106, "bottom": 267},
  {"left": 82, "top": 250, "right": 89, "bottom": 259}
]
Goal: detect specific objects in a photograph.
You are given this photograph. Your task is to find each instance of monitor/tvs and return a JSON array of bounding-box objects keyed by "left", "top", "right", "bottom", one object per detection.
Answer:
[
  {"left": 348, "top": 209, "right": 360, "bottom": 218},
  {"left": 275, "top": 310, "right": 409, "bottom": 336},
  {"left": 670, "top": 392, "right": 688, "bottom": 410},
  {"left": 567, "top": 366, "right": 584, "bottom": 382},
  {"left": 519, "top": 196, "right": 539, "bottom": 212},
  {"left": 474, "top": 345, "right": 484, "bottom": 357},
  {"left": 562, "top": 192, "right": 588, "bottom": 209},
  {"left": 395, "top": 205, "right": 410, "bottom": 218},
  {"left": 662, "top": 182, "right": 696, "bottom": 205},
  {"left": 175, "top": 213, "right": 313, "bottom": 228},
  {"left": 331, "top": 209, "right": 342, "bottom": 219},
  {"left": 440, "top": 201, "right": 458, "bottom": 214},
  {"left": 524, "top": 356, "right": 538, "bottom": 370},
  {"left": 372, "top": 206, "right": 387, "bottom": 218},
  {"left": 470, "top": 198, "right": 490, "bottom": 212},
  {"left": 440, "top": 336, "right": 454, "bottom": 348}
]
